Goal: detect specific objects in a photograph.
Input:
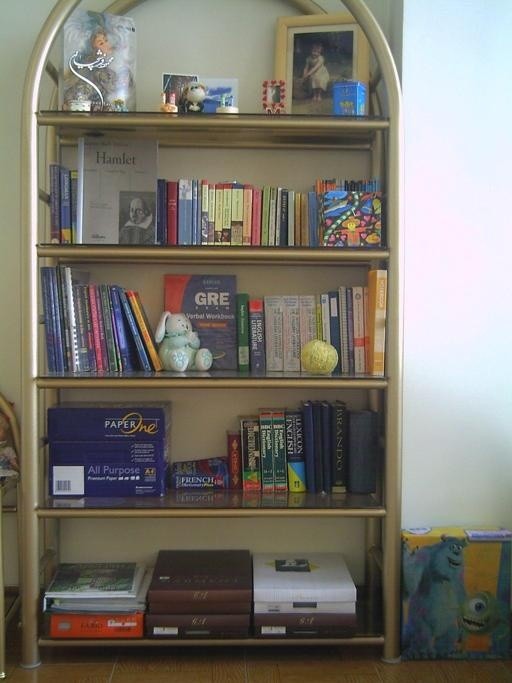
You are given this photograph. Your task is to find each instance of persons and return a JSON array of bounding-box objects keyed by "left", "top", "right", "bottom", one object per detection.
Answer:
[
  {"left": 62, "top": 20, "right": 134, "bottom": 113},
  {"left": 302, "top": 41, "right": 332, "bottom": 100}
]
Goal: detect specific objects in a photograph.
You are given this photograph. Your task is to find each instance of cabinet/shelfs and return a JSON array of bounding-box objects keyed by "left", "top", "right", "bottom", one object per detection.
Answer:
[
  {"left": 16, "top": 0, "right": 404, "bottom": 668},
  {"left": 0, "top": 394, "right": 21, "bottom": 683}
]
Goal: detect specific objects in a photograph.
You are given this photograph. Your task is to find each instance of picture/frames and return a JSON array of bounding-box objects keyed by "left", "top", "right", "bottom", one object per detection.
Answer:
[
  {"left": 263, "top": 80, "right": 286, "bottom": 117},
  {"left": 275, "top": 15, "right": 371, "bottom": 118}
]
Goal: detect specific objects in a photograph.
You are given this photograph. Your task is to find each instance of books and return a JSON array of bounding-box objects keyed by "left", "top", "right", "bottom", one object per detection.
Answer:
[
  {"left": 49, "top": 136, "right": 383, "bottom": 255},
  {"left": 49, "top": 398, "right": 378, "bottom": 495},
  {"left": 41, "top": 266, "right": 389, "bottom": 379}
]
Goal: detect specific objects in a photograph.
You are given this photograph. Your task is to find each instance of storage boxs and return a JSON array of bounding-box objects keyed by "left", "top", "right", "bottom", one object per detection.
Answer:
[{"left": 47, "top": 402, "right": 167, "bottom": 499}]
